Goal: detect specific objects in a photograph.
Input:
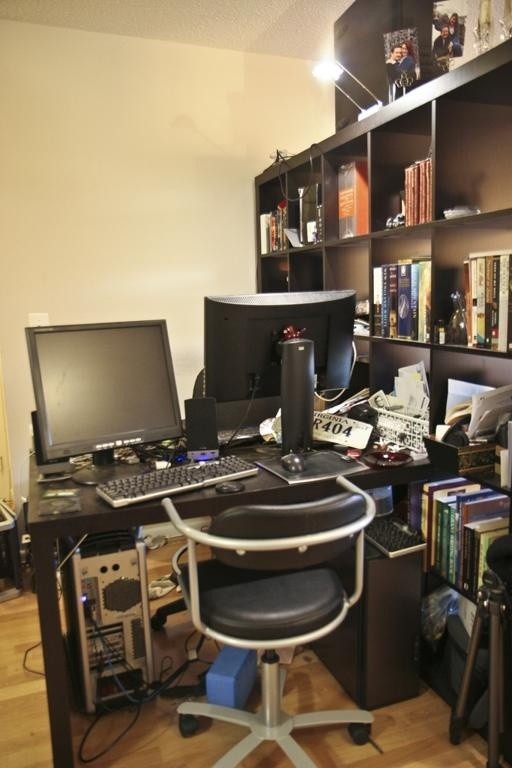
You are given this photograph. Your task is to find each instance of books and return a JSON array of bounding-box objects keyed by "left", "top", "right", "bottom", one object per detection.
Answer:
[
  {"left": 463, "top": 249, "right": 512, "bottom": 354},
  {"left": 408, "top": 476, "right": 511, "bottom": 596},
  {"left": 373, "top": 256, "right": 432, "bottom": 344},
  {"left": 404, "top": 159, "right": 433, "bottom": 226},
  {"left": 259, "top": 201, "right": 288, "bottom": 255}
]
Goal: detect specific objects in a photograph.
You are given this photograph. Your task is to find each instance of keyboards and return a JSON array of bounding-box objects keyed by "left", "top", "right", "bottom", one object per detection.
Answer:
[
  {"left": 96, "top": 452, "right": 259, "bottom": 510},
  {"left": 363, "top": 515, "right": 427, "bottom": 559}
]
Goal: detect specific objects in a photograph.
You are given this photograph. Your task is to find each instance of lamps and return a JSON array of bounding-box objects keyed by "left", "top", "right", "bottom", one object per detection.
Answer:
[{"left": 310, "top": 59, "right": 384, "bottom": 121}]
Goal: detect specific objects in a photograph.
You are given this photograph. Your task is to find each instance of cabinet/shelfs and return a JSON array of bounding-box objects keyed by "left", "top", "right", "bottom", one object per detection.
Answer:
[{"left": 253, "top": 36, "right": 512, "bottom": 434}]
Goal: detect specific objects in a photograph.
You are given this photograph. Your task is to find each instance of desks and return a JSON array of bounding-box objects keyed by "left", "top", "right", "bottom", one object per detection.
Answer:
[{"left": 27, "top": 422, "right": 431, "bottom": 768}]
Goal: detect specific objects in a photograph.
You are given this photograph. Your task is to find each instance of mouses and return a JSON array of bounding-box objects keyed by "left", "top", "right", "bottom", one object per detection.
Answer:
[{"left": 280, "top": 453, "right": 305, "bottom": 472}]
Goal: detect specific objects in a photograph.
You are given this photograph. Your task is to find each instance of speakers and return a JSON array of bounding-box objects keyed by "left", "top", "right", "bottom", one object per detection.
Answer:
[
  {"left": 184, "top": 397, "right": 220, "bottom": 460},
  {"left": 30, "top": 410, "right": 73, "bottom": 483}
]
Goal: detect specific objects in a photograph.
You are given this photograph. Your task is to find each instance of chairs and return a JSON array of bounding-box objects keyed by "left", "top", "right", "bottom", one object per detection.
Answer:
[{"left": 160, "top": 476, "right": 377, "bottom": 768}]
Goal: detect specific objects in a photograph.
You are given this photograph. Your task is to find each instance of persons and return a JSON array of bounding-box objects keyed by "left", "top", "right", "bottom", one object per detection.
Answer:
[
  {"left": 433, "top": 13, "right": 463, "bottom": 59},
  {"left": 387, "top": 41, "right": 416, "bottom": 84}
]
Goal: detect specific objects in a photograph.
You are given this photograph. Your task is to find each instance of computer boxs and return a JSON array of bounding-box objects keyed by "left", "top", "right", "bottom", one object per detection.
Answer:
[{"left": 56, "top": 528, "right": 155, "bottom": 715}]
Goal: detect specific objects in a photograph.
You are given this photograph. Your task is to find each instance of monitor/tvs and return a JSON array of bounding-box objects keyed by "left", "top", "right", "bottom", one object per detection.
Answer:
[
  {"left": 203, "top": 289, "right": 371, "bottom": 484},
  {"left": 23, "top": 319, "right": 184, "bottom": 488}
]
